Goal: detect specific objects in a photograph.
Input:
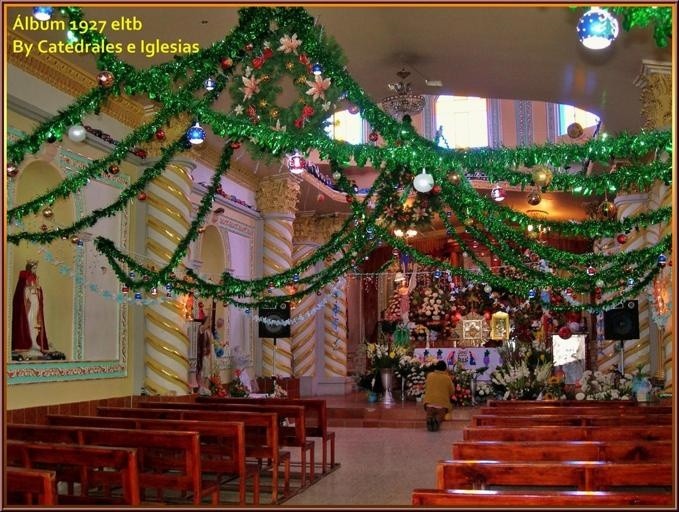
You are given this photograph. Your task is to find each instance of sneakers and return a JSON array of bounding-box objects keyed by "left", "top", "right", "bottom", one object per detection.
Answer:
[{"left": 424, "top": 416, "right": 440, "bottom": 432}]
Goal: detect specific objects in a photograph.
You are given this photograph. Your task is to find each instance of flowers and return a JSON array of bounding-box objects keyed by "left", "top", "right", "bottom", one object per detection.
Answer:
[
  {"left": 347, "top": 319, "right": 647, "bottom": 403},
  {"left": 209, "top": 367, "right": 228, "bottom": 399},
  {"left": 229, "top": 368, "right": 252, "bottom": 398}
]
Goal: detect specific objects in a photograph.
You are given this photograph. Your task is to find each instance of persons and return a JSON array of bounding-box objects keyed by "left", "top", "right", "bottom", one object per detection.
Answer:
[
  {"left": 197, "top": 316, "right": 215, "bottom": 393},
  {"left": 422, "top": 361, "right": 455, "bottom": 432}
]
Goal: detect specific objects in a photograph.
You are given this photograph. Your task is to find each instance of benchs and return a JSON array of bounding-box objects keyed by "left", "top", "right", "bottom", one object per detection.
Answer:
[
  {"left": 407, "top": 397, "right": 675, "bottom": 507},
  {"left": 6, "top": 394, "right": 337, "bottom": 506}
]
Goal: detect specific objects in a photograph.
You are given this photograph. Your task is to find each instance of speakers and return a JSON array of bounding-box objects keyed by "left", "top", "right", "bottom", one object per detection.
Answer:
[
  {"left": 604, "top": 300, "right": 640, "bottom": 340},
  {"left": 259, "top": 302, "right": 290, "bottom": 338}
]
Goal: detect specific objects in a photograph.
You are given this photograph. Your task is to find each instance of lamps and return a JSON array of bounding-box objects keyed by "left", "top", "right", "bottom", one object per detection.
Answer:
[{"left": 379, "top": 66, "right": 426, "bottom": 119}]
